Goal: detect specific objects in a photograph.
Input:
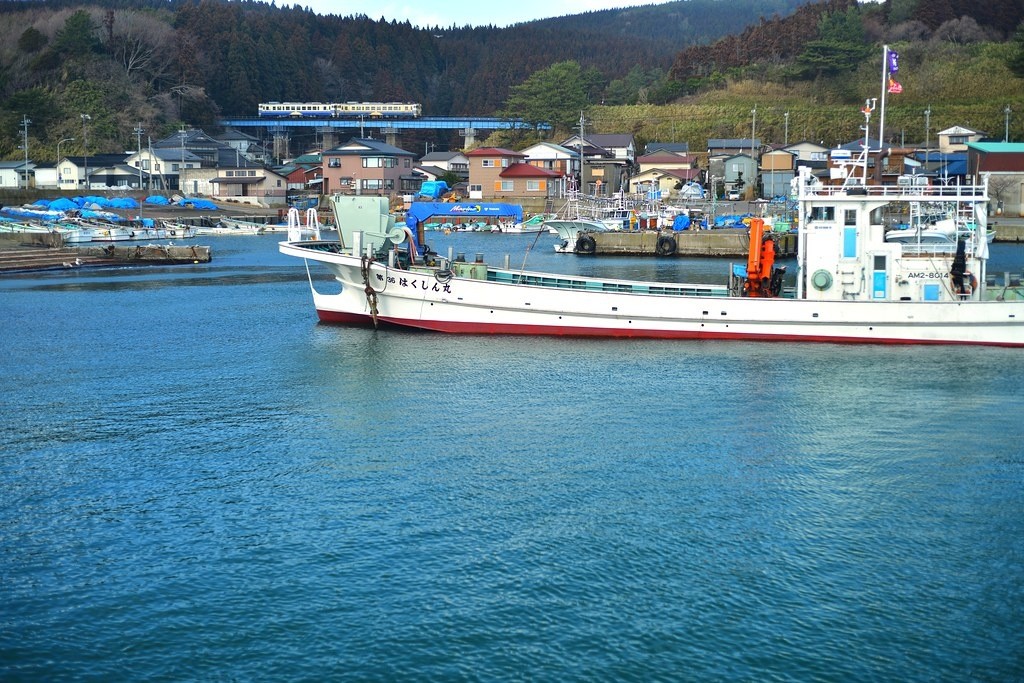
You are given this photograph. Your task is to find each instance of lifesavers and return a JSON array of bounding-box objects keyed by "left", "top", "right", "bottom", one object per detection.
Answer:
[
  {"left": 576, "top": 236, "right": 596, "bottom": 251},
  {"left": 130, "top": 231, "right": 134, "bottom": 237},
  {"left": 657, "top": 235, "right": 676, "bottom": 255},
  {"left": 171, "top": 231, "right": 175, "bottom": 236},
  {"left": 950, "top": 270, "right": 978, "bottom": 295}
]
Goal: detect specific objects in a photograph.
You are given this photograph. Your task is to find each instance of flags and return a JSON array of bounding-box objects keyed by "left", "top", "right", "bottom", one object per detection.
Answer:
[
  {"left": 888, "top": 78, "right": 902, "bottom": 94},
  {"left": 888, "top": 51, "right": 899, "bottom": 74}
]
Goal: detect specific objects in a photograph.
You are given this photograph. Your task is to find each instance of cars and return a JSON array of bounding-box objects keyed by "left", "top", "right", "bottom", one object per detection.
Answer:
[{"left": 728, "top": 189, "right": 740, "bottom": 201}]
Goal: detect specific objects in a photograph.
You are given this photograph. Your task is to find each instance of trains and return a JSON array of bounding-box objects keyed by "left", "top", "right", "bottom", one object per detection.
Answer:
[{"left": 257, "top": 99, "right": 423, "bottom": 121}]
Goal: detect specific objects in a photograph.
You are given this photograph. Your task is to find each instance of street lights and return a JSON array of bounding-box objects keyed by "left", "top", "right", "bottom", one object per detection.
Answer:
[
  {"left": 57, "top": 138, "right": 75, "bottom": 187},
  {"left": 761, "top": 143, "right": 774, "bottom": 198}
]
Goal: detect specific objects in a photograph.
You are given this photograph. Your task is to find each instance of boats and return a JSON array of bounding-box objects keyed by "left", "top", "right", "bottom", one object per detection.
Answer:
[
  {"left": 277, "top": 98, "right": 1024, "bottom": 347},
  {"left": 541, "top": 174, "right": 798, "bottom": 258},
  {"left": 422, "top": 210, "right": 558, "bottom": 234},
  {"left": 0, "top": 213, "right": 316, "bottom": 248}
]
